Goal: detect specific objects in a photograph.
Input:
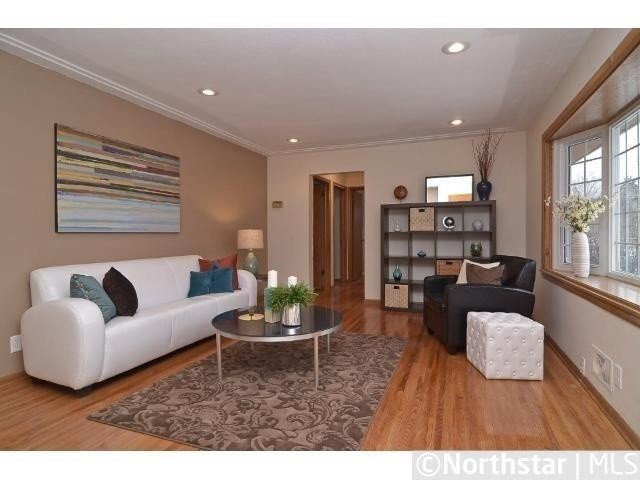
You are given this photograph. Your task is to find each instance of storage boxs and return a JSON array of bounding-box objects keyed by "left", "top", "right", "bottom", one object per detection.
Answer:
[
  {"left": 409, "top": 207, "right": 435, "bottom": 232},
  {"left": 436, "top": 259, "right": 463, "bottom": 276},
  {"left": 385, "top": 284, "right": 408, "bottom": 309}
]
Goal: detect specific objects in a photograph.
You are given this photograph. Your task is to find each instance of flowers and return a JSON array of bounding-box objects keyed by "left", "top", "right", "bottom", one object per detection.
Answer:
[{"left": 543, "top": 190, "right": 614, "bottom": 233}]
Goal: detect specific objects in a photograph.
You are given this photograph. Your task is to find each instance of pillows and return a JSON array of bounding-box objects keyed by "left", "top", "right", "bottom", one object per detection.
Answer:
[
  {"left": 188, "top": 270, "right": 212, "bottom": 297},
  {"left": 466, "top": 263, "right": 505, "bottom": 286},
  {"left": 211, "top": 266, "right": 233, "bottom": 292},
  {"left": 456, "top": 258, "right": 500, "bottom": 284},
  {"left": 103, "top": 267, "right": 138, "bottom": 316},
  {"left": 70, "top": 274, "right": 118, "bottom": 323},
  {"left": 198, "top": 255, "right": 241, "bottom": 290}
]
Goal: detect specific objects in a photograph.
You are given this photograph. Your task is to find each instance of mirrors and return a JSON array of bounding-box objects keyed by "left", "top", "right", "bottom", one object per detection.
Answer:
[{"left": 426, "top": 175, "right": 473, "bottom": 202}]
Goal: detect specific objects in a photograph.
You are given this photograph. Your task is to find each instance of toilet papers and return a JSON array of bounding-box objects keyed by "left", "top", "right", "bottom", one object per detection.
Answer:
[
  {"left": 288, "top": 276, "right": 297, "bottom": 287},
  {"left": 268, "top": 270, "right": 278, "bottom": 288}
]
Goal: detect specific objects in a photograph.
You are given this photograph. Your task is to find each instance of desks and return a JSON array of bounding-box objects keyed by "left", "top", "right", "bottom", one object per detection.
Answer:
[
  {"left": 212, "top": 306, "right": 343, "bottom": 390},
  {"left": 254, "top": 274, "right": 268, "bottom": 280}
]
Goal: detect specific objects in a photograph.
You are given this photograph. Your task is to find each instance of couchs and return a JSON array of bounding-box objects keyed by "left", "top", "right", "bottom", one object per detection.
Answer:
[
  {"left": 423, "top": 255, "right": 536, "bottom": 355},
  {"left": 21, "top": 255, "right": 258, "bottom": 398}
]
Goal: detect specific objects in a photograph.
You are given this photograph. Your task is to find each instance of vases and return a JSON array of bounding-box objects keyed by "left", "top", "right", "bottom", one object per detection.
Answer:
[
  {"left": 571, "top": 233, "right": 590, "bottom": 278},
  {"left": 393, "top": 263, "right": 402, "bottom": 282},
  {"left": 476, "top": 169, "right": 493, "bottom": 200},
  {"left": 471, "top": 217, "right": 484, "bottom": 230}
]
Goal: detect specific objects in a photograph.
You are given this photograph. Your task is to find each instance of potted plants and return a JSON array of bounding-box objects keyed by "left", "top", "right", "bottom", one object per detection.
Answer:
[{"left": 266, "top": 282, "right": 319, "bottom": 326}]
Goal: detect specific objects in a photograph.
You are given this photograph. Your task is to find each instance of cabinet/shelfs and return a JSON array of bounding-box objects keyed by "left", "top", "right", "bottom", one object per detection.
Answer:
[{"left": 380, "top": 200, "right": 496, "bottom": 313}]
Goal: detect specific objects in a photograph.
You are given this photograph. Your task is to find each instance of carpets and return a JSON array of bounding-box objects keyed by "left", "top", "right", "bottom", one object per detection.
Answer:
[{"left": 87, "top": 330, "right": 409, "bottom": 451}]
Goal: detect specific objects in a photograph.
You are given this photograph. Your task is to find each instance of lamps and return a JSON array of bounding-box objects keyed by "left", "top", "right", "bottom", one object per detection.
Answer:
[{"left": 237, "top": 228, "right": 265, "bottom": 275}]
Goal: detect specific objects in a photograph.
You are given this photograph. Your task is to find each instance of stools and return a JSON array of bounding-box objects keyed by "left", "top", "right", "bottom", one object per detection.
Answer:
[{"left": 467, "top": 311, "right": 545, "bottom": 381}]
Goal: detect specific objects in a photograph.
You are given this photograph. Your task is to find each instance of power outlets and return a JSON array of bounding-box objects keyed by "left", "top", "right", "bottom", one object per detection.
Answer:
[
  {"left": 613, "top": 364, "right": 623, "bottom": 389},
  {"left": 579, "top": 358, "right": 586, "bottom": 376},
  {"left": 11, "top": 334, "right": 21, "bottom": 352}
]
